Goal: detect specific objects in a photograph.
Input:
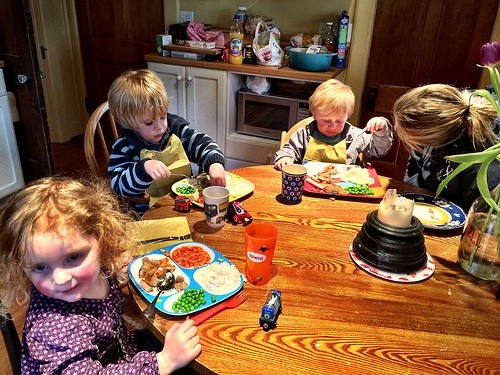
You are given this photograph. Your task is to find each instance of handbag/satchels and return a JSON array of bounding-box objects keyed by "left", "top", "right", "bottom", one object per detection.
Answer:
[{"left": 252, "top": 20, "right": 285, "bottom": 67}]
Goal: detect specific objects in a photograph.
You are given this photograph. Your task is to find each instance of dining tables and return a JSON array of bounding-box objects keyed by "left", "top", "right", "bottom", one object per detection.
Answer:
[{"left": 125, "top": 164, "right": 500, "bottom": 375}]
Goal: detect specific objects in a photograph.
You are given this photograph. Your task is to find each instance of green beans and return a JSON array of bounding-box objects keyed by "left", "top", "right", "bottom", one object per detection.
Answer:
[
  {"left": 176, "top": 186, "right": 196, "bottom": 194},
  {"left": 346, "top": 183, "right": 374, "bottom": 195},
  {"left": 172, "top": 288, "right": 216, "bottom": 313}
]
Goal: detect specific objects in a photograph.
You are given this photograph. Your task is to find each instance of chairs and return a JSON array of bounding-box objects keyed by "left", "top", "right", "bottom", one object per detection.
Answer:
[
  {"left": 84, "top": 101, "right": 124, "bottom": 181},
  {"left": 0, "top": 299, "right": 22, "bottom": 375},
  {"left": 280, "top": 115, "right": 364, "bottom": 167}
]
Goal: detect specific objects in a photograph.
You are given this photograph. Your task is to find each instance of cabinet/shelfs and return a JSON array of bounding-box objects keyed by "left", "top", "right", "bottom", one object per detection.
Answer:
[{"left": 145, "top": 62, "right": 345, "bottom": 171}]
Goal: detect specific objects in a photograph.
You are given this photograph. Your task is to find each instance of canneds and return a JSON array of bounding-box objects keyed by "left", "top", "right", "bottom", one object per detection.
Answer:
[{"left": 242, "top": 45, "right": 255, "bottom": 64}]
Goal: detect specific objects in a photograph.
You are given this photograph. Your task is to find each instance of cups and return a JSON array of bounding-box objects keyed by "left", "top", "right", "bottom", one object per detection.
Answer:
[
  {"left": 244, "top": 223, "right": 278, "bottom": 286},
  {"left": 203, "top": 186, "right": 230, "bottom": 227},
  {"left": 282, "top": 164, "right": 307, "bottom": 203}
]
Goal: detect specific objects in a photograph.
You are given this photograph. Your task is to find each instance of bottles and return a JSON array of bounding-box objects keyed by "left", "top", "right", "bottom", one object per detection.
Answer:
[
  {"left": 322, "top": 21, "right": 338, "bottom": 69},
  {"left": 243, "top": 44, "right": 255, "bottom": 64},
  {"left": 229, "top": 15, "right": 243, "bottom": 64},
  {"left": 235, "top": 7, "right": 248, "bottom": 28}
]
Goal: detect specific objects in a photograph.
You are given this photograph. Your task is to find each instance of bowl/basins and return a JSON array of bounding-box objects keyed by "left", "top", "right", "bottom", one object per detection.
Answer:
[{"left": 285, "top": 46, "right": 337, "bottom": 72}]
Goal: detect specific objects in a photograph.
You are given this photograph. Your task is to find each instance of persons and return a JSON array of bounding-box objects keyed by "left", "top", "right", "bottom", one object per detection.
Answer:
[
  {"left": 392, "top": 83, "right": 500, "bottom": 214},
  {"left": 0, "top": 177, "right": 202, "bottom": 375},
  {"left": 108, "top": 70, "right": 226, "bottom": 217},
  {"left": 272, "top": 79, "right": 393, "bottom": 170}
]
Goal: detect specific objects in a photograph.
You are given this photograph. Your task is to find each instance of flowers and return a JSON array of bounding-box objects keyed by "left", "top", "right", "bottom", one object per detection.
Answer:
[{"left": 435, "top": 41, "right": 500, "bottom": 267}]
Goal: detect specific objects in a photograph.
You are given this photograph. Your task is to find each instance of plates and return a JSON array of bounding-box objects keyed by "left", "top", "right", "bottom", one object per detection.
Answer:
[
  {"left": 129, "top": 241, "right": 243, "bottom": 316},
  {"left": 171, "top": 170, "right": 255, "bottom": 209},
  {"left": 396, "top": 193, "right": 467, "bottom": 230},
  {"left": 304, "top": 164, "right": 385, "bottom": 199}
]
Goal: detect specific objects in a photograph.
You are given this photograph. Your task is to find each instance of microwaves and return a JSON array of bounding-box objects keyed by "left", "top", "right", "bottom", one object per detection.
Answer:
[{"left": 235, "top": 82, "right": 313, "bottom": 142}]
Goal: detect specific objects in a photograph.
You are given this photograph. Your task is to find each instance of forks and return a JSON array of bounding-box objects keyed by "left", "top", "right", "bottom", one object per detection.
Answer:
[{"left": 189, "top": 290, "right": 249, "bottom": 326}]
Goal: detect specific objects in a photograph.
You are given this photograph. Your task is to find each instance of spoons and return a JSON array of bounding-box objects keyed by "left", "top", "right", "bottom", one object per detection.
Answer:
[{"left": 140, "top": 271, "right": 175, "bottom": 317}]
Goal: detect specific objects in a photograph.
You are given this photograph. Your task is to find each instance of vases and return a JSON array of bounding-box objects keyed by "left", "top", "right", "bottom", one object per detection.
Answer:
[{"left": 457, "top": 182, "right": 500, "bottom": 281}]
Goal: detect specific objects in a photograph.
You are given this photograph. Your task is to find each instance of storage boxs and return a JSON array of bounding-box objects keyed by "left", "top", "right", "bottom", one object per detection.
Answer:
[{"left": 156, "top": 34, "right": 173, "bottom": 56}]
